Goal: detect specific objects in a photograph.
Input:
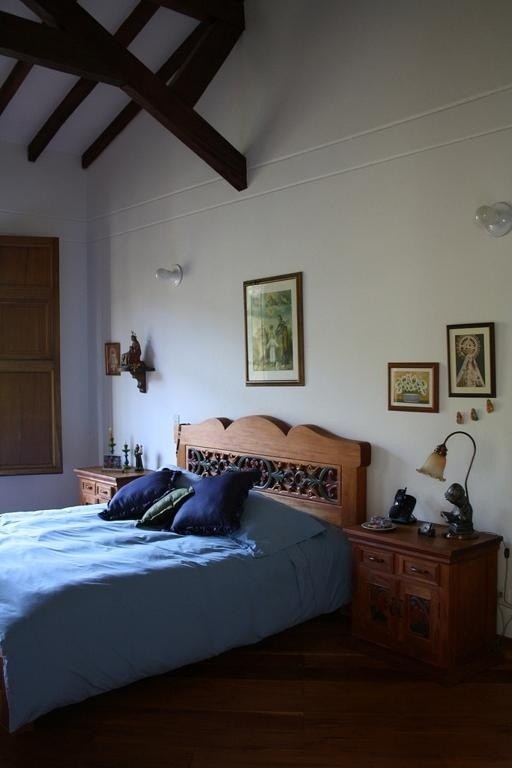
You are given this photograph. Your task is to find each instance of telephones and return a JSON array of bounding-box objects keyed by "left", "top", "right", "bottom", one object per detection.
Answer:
[{"left": 390, "top": 486, "right": 416, "bottom": 526}]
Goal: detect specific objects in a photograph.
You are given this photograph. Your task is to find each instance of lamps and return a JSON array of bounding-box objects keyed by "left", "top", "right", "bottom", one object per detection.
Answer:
[
  {"left": 416, "top": 432, "right": 481, "bottom": 541},
  {"left": 153, "top": 263, "right": 183, "bottom": 289},
  {"left": 472, "top": 201, "right": 512, "bottom": 236}
]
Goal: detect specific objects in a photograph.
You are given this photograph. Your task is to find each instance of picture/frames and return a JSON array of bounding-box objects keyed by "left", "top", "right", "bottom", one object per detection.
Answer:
[
  {"left": 242, "top": 272, "right": 305, "bottom": 386},
  {"left": 105, "top": 342, "right": 120, "bottom": 376},
  {"left": 386, "top": 361, "right": 441, "bottom": 413},
  {"left": 445, "top": 323, "right": 498, "bottom": 399}
]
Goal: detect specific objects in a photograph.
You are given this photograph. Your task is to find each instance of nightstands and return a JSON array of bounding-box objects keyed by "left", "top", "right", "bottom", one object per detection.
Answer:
[
  {"left": 73, "top": 463, "right": 157, "bottom": 505},
  {"left": 342, "top": 519, "right": 505, "bottom": 668}
]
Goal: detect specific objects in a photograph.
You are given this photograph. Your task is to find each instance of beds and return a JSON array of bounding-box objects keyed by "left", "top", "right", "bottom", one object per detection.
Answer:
[{"left": 0, "top": 417, "right": 373, "bottom": 740}]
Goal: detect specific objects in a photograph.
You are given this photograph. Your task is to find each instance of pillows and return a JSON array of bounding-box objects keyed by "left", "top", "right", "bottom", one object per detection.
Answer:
[
  {"left": 138, "top": 489, "right": 196, "bottom": 527},
  {"left": 171, "top": 470, "right": 262, "bottom": 535},
  {"left": 100, "top": 467, "right": 181, "bottom": 521}
]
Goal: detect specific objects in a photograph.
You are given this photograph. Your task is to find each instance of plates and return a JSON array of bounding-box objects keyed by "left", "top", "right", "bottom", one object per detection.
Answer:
[{"left": 362, "top": 522, "right": 398, "bottom": 531}]
